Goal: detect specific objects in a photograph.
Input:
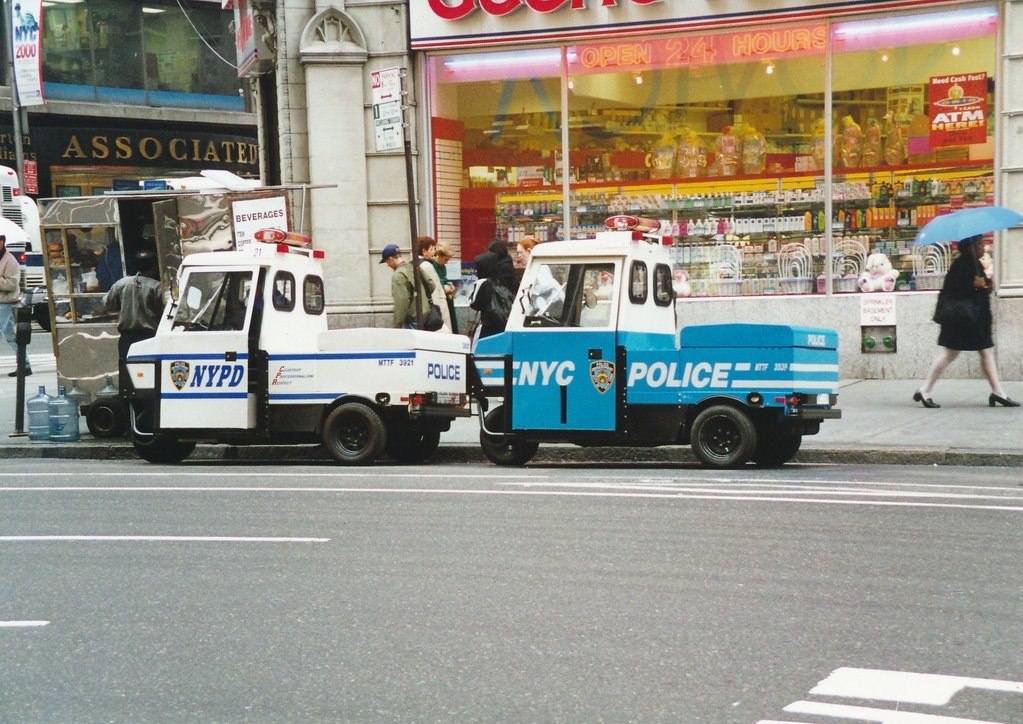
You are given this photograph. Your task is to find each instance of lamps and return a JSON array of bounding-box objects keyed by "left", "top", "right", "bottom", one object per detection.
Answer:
[{"left": 835, "top": 7, "right": 998, "bottom": 38}]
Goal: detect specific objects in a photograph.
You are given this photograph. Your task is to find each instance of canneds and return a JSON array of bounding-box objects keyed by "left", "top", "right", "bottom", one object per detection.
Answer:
[{"left": 496, "top": 201, "right": 564, "bottom": 216}]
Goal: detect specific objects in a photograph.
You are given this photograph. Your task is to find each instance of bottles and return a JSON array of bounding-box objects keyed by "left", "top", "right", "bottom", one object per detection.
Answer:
[
  {"left": 48, "top": 386, "right": 79, "bottom": 441},
  {"left": 96, "top": 377, "right": 119, "bottom": 398},
  {"left": 691, "top": 272, "right": 776, "bottom": 296},
  {"left": 497, "top": 166, "right": 939, "bottom": 263},
  {"left": 652, "top": 123, "right": 767, "bottom": 180},
  {"left": 27, "top": 386, "right": 52, "bottom": 439},
  {"left": 814, "top": 111, "right": 902, "bottom": 168},
  {"left": 66, "top": 380, "right": 91, "bottom": 404}
]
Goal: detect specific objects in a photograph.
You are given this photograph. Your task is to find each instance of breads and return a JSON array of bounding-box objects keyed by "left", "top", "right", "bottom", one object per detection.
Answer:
[
  {"left": 811, "top": 120, "right": 906, "bottom": 168},
  {"left": 650, "top": 129, "right": 768, "bottom": 179}
]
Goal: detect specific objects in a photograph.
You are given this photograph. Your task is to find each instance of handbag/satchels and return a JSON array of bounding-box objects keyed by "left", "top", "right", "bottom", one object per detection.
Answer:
[
  {"left": 425, "top": 305, "right": 444, "bottom": 331},
  {"left": 482, "top": 278, "right": 512, "bottom": 328},
  {"left": 931, "top": 287, "right": 980, "bottom": 322}
]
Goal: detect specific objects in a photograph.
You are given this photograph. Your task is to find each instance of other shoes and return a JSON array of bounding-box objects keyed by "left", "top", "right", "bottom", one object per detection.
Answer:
[{"left": 9, "top": 367, "right": 32, "bottom": 377}]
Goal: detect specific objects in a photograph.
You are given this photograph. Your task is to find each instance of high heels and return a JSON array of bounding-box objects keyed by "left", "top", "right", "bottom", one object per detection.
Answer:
[
  {"left": 914, "top": 390, "right": 940, "bottom": 408},
  {"left": 988, "top": 393, "right": 1021, "bottom": 407}
]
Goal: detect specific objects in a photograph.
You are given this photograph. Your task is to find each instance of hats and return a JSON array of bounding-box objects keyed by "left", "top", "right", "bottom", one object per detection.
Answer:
[
  {"left": 379, "top": 244, "right": 400, "bottom": 263},
  {"left": 136, "top": 251, "right": 157, "bottom": 272}
]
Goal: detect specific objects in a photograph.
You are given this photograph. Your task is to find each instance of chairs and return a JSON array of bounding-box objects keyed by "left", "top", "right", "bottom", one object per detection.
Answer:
[{"left": 444, "top": 46, "right": 577, "bottom": 68}]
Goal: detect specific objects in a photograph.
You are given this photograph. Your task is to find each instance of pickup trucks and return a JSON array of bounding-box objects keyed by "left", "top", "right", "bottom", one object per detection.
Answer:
[
  {"left": 472, "top": 215, "right": 842, "bottom": 470},
  {"left": 124, "top": 225, "right": 475, "bottom": 465}
]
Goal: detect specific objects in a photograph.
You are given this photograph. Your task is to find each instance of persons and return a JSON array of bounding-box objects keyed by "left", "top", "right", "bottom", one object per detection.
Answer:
[
  {"left": 379, "top": 245, "right": 436, "bottom": 330},
  {"left": 914, "top": 233, "right": 1020, "bottom": 408},
  {"left": 470, "top": 239, "right": 566, "bottom": 340},
  {"left": 233, "top": 271, "right": 290, "bottom": 332},
  {"left": 105, "top": 251, "right": 165, "bottom": 440},
  {"left": 0, "top": 235, "right": 32, "bottom": 376},
  {"left": 410, "top": 236, "right": 457, "bottom": 334}
]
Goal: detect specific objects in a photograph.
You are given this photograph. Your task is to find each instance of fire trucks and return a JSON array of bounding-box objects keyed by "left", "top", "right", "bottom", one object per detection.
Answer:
[{"left": 0, "top": 165, "right": 57, "bottom": 332}]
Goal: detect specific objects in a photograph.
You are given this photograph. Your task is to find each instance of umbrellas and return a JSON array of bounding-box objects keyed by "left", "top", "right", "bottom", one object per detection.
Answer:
[
  {"left": 915, "top": 206, "right": 1023, "bottom": 278},
  {"left": 0, "top": 218, "right": 30, "bottom": 244}
]
